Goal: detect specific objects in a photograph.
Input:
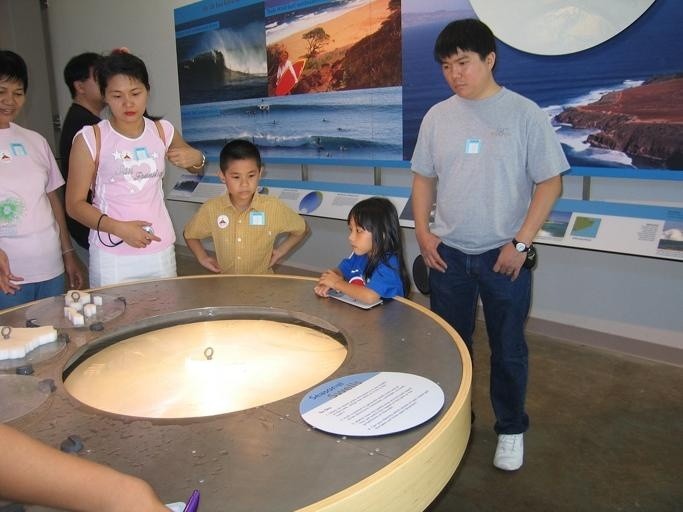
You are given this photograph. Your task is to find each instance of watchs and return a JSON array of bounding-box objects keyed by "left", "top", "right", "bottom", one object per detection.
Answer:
[{"left": 512, "top": 239, "right": 534, "bottom": 252}]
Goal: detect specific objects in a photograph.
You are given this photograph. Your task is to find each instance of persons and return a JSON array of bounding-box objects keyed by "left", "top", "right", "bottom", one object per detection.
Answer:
[
  {"left": 0, "top": 421, "right": 178, "bottom": 512},
  {"left": 182, "top": 140, "right": 311, "bottom": 274},
  {"left": 276, "top": 51, "right": 298, "bottom": 95},
  {"left": 58, "top": 51, "right": 108, "bottom": 271},
  {"left": 410, "top": 18, "right": 572, "bottom": 473},
  {"left": 0, "top": 49, "right": 86, "bottom": 312},
  {"left": 64, "top": 49, "right": 206, "bottom": 291},
  {"left": 314, "top": 196, "right": 410, "bottom": 304}
]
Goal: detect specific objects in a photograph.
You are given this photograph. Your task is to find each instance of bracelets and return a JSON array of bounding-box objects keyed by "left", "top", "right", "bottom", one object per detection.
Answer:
[
  {"left": 193, "top": 154, "right": 205, "bottom": 170},
  {"left": 62, "top": 248, "right": 76, "bottom": 254}
]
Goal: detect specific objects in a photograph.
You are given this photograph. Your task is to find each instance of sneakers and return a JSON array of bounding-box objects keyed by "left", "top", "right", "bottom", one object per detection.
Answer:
[{"left": 493, "top": 433, "right": 524, "bottom": 471}]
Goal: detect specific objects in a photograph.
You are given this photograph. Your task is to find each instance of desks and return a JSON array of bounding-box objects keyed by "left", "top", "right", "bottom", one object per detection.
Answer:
[{"left": 0, "top": 273, "right": 476, "bottom": 511}]
